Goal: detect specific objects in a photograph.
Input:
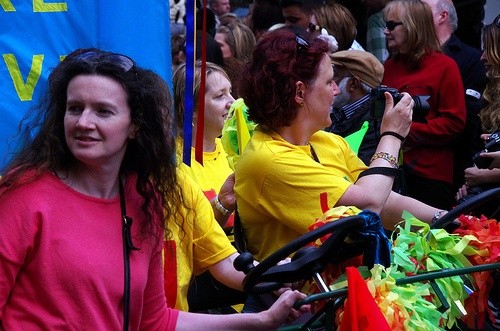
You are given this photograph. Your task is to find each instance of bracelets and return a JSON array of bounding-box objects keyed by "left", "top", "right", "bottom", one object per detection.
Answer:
[
  {"left": 213, "top": 194, "right": 233, "bottom": 219},
  {"left": 370, "top": 153, "right": 397, "bottom": 167},
  {"left": 380, "top": 131, "right": 405, "bottom": 142},
  {"left": 430, "top": 209, "right": 445, "bottom": 225}
]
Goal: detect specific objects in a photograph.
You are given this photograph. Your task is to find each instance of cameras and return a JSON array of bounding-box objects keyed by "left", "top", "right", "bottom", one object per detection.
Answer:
[
  {"left": 370, "top": 85, "right": 432, "bottom": 124},
  {"left": 473, "top": 130, "right": 500, "bottom": 169}
]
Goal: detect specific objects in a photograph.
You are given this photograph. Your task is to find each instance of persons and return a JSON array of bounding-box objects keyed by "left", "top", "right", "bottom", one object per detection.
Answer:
[
  {"left": 171, "top": 0, "right": 500, "bottom": 331},
  {"left": 0, "top": 48, "right": 310, "bottom": 331},
  {"left": 133, "top": 69, "right": 306, "bottom": 312}
]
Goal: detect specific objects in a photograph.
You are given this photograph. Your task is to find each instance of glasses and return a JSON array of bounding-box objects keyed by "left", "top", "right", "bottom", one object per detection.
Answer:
[
  {"left": 385, "top": 20, "right": 403, "bottom": 30},
  {"left": 308, "top": 23, "right": 319, "bottom": 32},
  {"left": 72, "top": 51, "right": 136, "bottom": 72},
  {"left": 294, "top": 34, "right": 310, "bottom": 61}
]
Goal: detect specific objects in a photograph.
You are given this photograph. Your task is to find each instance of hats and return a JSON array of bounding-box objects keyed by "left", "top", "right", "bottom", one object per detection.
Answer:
[{"left": 329, "top": 50, "right": 385, "bottom": 89}]
